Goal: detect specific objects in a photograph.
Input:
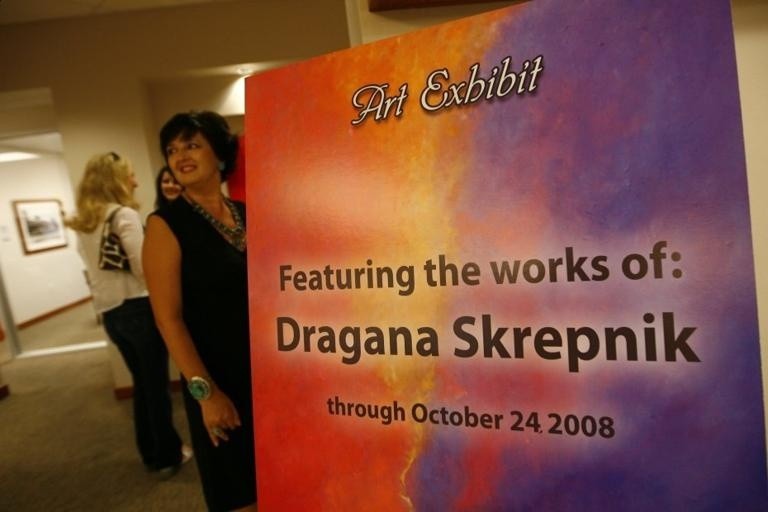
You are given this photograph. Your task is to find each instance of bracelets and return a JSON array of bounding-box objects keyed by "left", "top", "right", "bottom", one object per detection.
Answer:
[{"left": 187, "top": 375, "right": 215, "bottom": 400}]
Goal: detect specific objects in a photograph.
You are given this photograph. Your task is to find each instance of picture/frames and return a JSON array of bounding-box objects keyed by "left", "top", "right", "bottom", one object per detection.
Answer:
[{"left": 11, "top": 198, "right": 68, "bottom": 256}]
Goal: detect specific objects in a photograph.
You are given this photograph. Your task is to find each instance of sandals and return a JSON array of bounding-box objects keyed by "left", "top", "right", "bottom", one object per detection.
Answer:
[{"left": 145, "top": 448, "right": 192, "bottom": 481}]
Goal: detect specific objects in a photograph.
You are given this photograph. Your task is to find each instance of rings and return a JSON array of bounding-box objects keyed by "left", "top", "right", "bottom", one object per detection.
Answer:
[{"left": 212, "top": 426, "right": 222, "bottom": 436}]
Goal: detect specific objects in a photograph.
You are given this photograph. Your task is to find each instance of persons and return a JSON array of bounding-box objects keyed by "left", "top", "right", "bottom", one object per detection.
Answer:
[
  {"left": 62, "top": 148, "right": 193, "bottom": 482},
  {"left": 154, "top": 165, "right": 183, "bottom": 212},
  {"left": 142, "top": 109, "right": 258, "bottom": 511}
]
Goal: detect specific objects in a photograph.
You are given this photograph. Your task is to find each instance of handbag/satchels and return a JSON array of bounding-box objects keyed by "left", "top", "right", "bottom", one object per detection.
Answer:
[{"left": 97, "top": 205, "right": 129, "bottom": 272}]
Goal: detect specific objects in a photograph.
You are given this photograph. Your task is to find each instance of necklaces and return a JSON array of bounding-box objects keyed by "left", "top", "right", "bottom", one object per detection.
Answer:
[{"left": 186, "top": 190, "right": 246, "bottom": 250}]
